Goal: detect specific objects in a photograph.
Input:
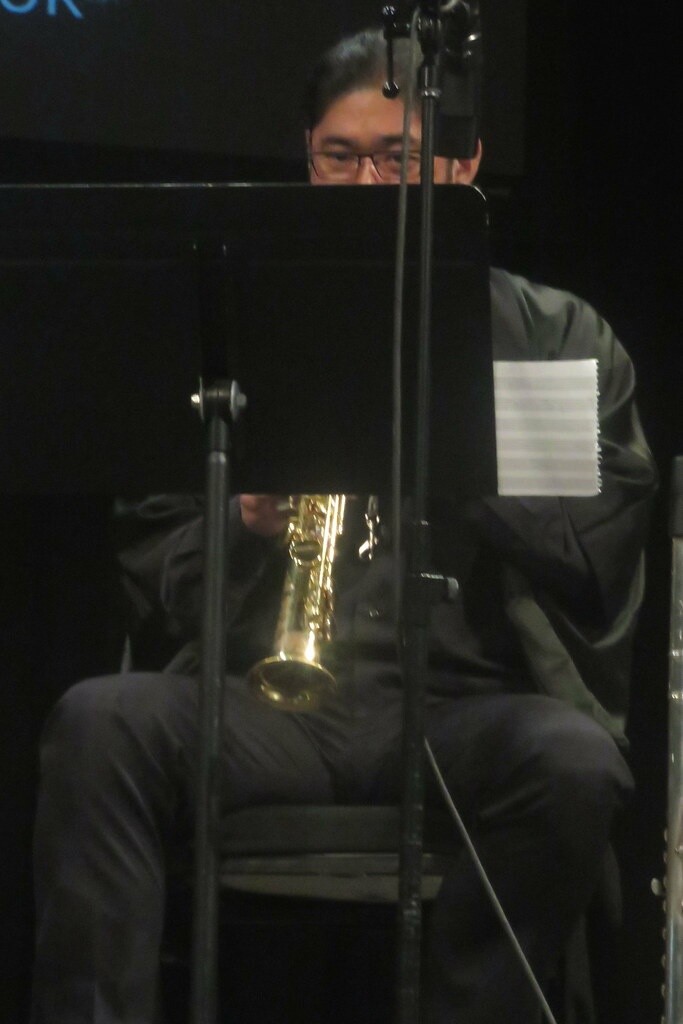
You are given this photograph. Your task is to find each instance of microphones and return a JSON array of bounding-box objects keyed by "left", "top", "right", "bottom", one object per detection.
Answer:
[{"left": 423, "top": 0, "right": 484, "bottom": 160}]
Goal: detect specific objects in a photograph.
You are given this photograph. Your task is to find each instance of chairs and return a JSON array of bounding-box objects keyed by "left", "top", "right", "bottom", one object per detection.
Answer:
[{"left": 172, "top": 796, "right": 446, "bottom": 1024}]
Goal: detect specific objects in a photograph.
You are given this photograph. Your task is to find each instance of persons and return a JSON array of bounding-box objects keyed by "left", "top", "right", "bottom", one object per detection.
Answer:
[{"left": 30, "top": 29, "right": 658, "bottom": 1024}]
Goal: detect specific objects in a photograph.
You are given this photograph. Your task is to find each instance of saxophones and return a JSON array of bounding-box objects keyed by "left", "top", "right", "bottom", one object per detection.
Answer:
[{"left": 244, "top": 495, "right": 348, "bottom": 715}]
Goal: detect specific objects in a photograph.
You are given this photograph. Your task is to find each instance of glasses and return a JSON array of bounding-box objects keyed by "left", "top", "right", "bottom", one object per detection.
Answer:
[{"left": 308, "top": 131, "right": 422, "bottom": 182}]
